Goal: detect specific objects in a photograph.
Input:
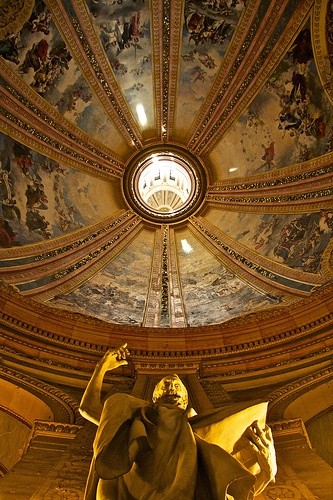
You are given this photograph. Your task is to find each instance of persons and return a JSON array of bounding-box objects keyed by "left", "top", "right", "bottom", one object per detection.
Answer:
[{"left": 78, "top": 341, "right": 277, "bottom": 500}]
